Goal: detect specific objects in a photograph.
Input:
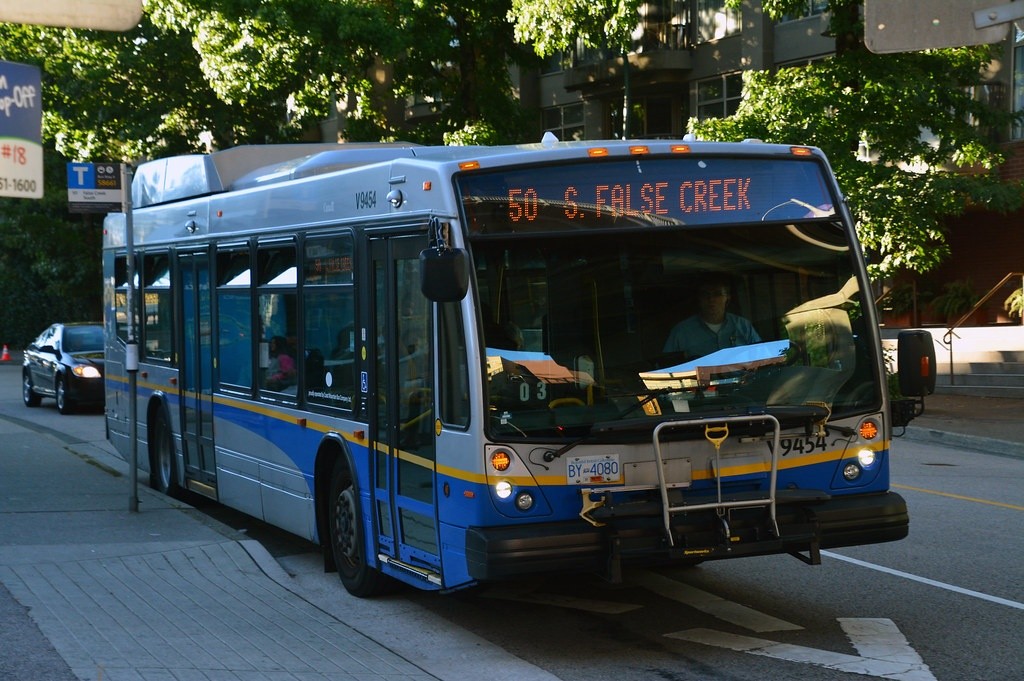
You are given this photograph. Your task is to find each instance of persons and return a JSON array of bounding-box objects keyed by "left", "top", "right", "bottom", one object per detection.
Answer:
[
  {"left": 263, "top": 292, "right": 543, "bottom": 450},
  {"left": 662, "top": 276, "right": 764, "bottom": 370},
  {"left": 65, "top": 334, "right": 86, "bottom": 352}
]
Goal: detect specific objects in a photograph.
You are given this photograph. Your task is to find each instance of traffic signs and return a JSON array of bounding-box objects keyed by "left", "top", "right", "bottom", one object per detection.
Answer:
[{"left": 1, "top": 61, "right": 47, "bottom": 202}]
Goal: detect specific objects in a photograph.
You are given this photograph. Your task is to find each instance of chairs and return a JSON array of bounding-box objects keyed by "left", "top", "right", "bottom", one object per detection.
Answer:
[{"left": 132, "top": 309, "right": 643, "bottom": 447}]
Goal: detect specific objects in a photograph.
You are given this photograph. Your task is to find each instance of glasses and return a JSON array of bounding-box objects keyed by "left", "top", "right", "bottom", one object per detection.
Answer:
[{"left": 700, "top": 291, "right": 727, "bottom": 298}]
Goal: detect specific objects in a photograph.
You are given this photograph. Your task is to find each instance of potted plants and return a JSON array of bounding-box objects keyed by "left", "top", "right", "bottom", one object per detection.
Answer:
[
  {"left": 933, "top": 279, "right": 989, "bottom": 326},
  {"left": 882, "top": 283, "right": 922, "bottom": 329}
]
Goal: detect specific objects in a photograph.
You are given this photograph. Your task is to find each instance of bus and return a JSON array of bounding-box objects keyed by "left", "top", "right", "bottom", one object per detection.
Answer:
[{"left": 100, "top": 117, "right": 939, "bottom": 620}]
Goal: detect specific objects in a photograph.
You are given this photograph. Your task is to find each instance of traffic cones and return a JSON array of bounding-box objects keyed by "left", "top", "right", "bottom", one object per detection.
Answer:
[{"left": 0, "top": 344, "right": 12, "bottom": 362}]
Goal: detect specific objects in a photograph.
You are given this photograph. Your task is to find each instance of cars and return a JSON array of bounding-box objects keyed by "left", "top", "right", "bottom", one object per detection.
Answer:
[{"left": 20, "top": 320, "right": 112, "bottom": 415}]
